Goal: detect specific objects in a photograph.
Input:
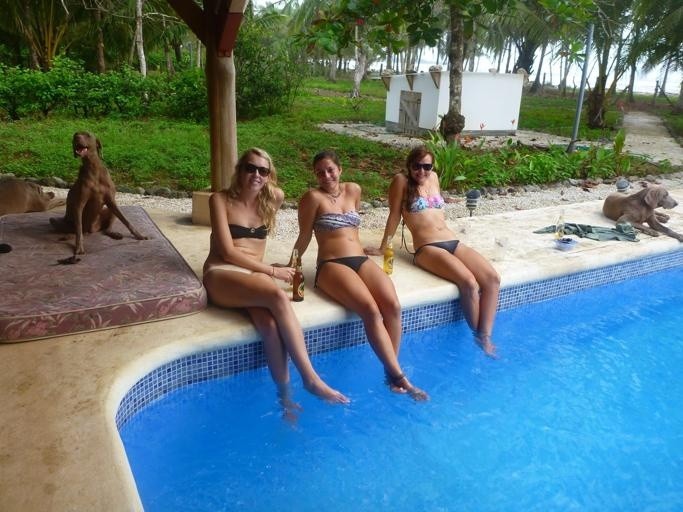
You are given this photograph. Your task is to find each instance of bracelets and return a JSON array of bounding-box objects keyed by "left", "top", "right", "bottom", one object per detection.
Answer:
[{"left": 271, "top": 265, "right": 274, "bottom": 276}]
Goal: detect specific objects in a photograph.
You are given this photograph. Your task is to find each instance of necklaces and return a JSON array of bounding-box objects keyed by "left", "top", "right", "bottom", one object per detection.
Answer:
[{"left": 321, "top": 188, "right": 342, "bottom": 199}]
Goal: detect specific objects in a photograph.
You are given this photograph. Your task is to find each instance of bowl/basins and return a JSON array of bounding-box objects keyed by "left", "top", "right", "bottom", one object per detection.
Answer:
[{"left": 554, "top": 239, "right": 577, "bottom": 251}]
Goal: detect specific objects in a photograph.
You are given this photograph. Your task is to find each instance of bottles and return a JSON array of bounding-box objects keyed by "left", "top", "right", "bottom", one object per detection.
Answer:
[
  {"left": 554, "top": 209, "right": 565, "bottom": 239},
  {"left": 383, "top": 234, "right": 393, "bottom": 274},
  {"left": 288, "top": 248, "right": 305, "bottom": 302}
]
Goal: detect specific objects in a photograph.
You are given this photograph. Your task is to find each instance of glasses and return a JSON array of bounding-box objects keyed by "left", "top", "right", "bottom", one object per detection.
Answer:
[
  {"left": 245, "top": 163, "right": 269, "bottom": 177},
  {"left": 412, "top": 163, "right": 432, "bottom": 171}
]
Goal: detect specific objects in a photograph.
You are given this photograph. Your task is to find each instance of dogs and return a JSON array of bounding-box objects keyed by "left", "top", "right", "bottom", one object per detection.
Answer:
[
  {"left": 49, "top": 131, "right": 147, "bottom": 254},
  {"left": 0, "top": 180, "right": 66, "bottom": 216},
  {"left": 602, "top": 186, "right": 683, "bottom": 242}
]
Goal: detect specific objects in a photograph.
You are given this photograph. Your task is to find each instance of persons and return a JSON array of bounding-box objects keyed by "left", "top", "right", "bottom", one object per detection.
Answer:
[
  {"left": 199, "top": 147, "right": 352, "bottom": 424},
  {"left": 363, "top": 145, "right": 499, "bottom": 360},
  {"left": 267, "top": 150, "right": 429, "bottom": 404}
]
